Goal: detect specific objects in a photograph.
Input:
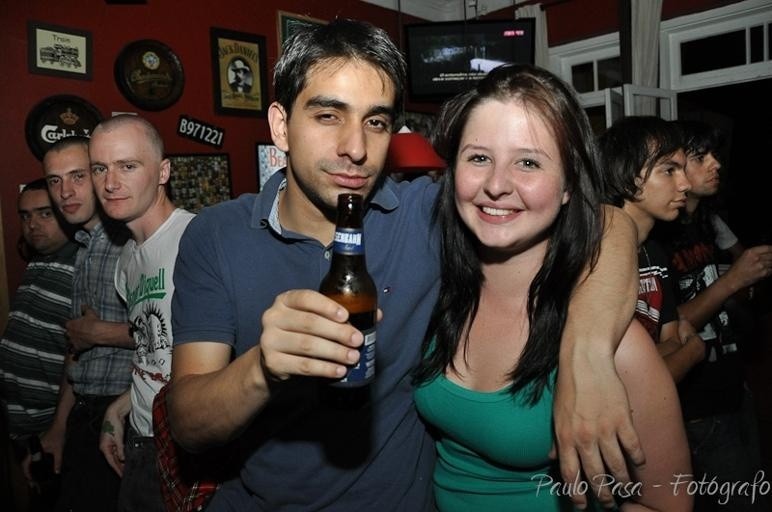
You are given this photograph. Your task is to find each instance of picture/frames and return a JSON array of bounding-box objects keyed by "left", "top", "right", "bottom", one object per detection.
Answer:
[
  {"left": 210, "top": 27, "right": 268, "bottom": 117},
  {"left": 255, "top": 141, "right": 287, "bottom": 193},
  {"left": 23, "top": 96, "right": 103, "bottom": 162},
  {"left": 25, "top": 20, "right": 94, "bottom": 81},
  {"left": 276, "top": 9, "right": 329, "bottom": 62},
  {"left": 114, "top": 40, "right": 185, "bottom": 112},
  {"left": 166, "top": 151, "right": 234, "bottom": 214}
]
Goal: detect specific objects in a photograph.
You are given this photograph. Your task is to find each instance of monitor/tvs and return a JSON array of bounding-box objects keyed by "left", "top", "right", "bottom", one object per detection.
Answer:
[{"left": 401, "top": 17, "right": 536, "bottom": 102}]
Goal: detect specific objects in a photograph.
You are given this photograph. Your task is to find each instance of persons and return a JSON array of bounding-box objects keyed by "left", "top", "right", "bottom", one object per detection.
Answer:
[
  {"left": 231, "top": 60, "right": 252, "bottom": 93},
  {"left": 90, "top": 116, "right": 196, "bottom": 512},
  {"left": 415, "top": 64, "right": 691, "bottom": 512},
  {"left": 166, "top": 20, "right": 645, "bottom": 512},
  {"left": 22, "top": 137, "right": 136, "bottom": 510},
  {"left": 597, "top": 115, "right": 707, "bottom": 481},
  {"left": 667, "top": 120, "right": 768, "bottom": 512},
  {"left": 0, "top": 180, "right": 81, "bottom": 512}
]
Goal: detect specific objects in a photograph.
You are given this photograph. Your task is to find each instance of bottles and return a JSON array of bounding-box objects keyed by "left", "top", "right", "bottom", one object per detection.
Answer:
[
  {"left": 23, "top": 431, "right": 56, "bottom": 487},
  {"left": 316, "top": 193, "right": 380, "bottom": 389}
]
